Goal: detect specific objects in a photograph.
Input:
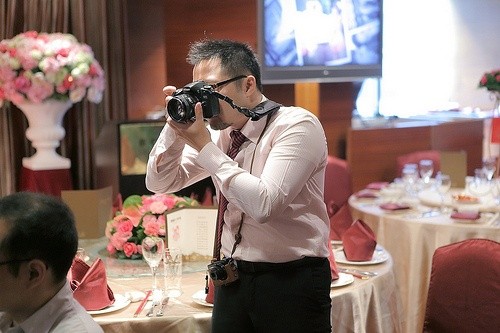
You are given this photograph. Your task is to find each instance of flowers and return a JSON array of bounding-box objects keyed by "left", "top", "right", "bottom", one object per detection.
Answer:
[
  {"left": 1, "top": 30, "right": 105, "bottom": 106},
  {"left": 107, "top": 195, "right": 197, "bottom": 257},
  {"left": 479, "top": 69, "right": 499, "bottom": 93}
]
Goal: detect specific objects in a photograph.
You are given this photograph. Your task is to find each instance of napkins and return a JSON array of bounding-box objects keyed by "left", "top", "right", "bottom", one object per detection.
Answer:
[
  {"left": 452, "top": 212, "right": 481, "bottom": 220},
  {"left": 69, "top": 258, "right": 115, "bottom": 308},
  {"left": 322, "top": 201, "right": 378, "bottom": 283},
  {"left": 380, "top": 202, "right": 409, "bottom": 211},
  {"left": 367, "top": 181, "right": 388, "bottom": 189},
  {"left": 203, "top": 271, "right": 217, "bottom": 302},
  {"left": 353, "top": 192, "right": 377, "bottom": 198}
]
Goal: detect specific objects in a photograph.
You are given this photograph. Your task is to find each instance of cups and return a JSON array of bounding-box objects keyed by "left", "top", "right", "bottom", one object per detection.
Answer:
[{"left": 164, "top": 247, "right": 182, "bottom": 295}]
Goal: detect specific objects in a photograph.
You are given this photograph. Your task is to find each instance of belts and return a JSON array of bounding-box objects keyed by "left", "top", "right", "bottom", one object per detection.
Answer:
[{"left": 236, "top": 258, "right": 277, "bottom": 274}]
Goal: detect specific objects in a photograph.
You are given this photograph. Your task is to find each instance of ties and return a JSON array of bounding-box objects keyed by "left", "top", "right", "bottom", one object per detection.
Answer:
[{"left": 212, "top": 129, "right": 248, "bottom": 260}]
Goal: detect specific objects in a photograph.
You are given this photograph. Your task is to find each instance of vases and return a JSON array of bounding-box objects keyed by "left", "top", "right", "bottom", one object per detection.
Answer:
[{"left": 18, "top": 97, "right": 75, "bottom": 171}]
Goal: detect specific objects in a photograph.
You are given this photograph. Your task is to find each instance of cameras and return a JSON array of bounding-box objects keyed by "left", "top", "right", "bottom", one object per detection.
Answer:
[{"left": 166, "top": 80, "right": 223, "bottom": 123}]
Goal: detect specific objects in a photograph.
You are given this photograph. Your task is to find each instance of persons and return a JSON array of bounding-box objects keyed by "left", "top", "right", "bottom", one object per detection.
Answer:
[
  {"left": 0, "top": 192, "right": 106, "bottom": 332},
  {"left": 146, "top": 44, "right": 334, "bottom": 333}
]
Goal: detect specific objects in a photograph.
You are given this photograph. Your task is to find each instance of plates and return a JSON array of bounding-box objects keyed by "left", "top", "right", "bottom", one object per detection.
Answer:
[
  {"left": 331, "top": 240, "right": 343, "bottom": 245},
  {"left": 455, "top": 218, "right": 484, "bottom": 223},
  {"left": 334, "top": 250, "right": 388, "bottom": 265},
  {"left": 330, "top": 272, "right": 354, "bottom": 287},
  {"left": 86, "top": 293, "right": 131, "bottom": 314},
  {"left": 192, "top": 290, "right": 214, "bottom": 308}
]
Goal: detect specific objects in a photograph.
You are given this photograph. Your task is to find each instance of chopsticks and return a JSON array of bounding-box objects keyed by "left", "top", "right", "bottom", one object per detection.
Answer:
[{"left": 134, "top": 290, "right": 152, "bottom": 314}]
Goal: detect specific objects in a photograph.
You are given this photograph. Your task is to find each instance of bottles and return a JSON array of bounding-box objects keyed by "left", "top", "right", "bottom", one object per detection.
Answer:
[{"left": 75, "top": 248, "right": 93, "bottom": 266}]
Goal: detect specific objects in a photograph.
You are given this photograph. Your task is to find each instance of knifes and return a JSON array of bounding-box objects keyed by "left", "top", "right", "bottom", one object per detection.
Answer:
[{"left": 156, "top": 297, "right": 169, "bottom": 316}]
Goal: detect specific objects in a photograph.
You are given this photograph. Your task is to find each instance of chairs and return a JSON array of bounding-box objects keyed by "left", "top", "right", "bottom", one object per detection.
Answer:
[
  {"left": 399, "top": 150, "right": 442, "bottom": 179},
  {"left": 420, "top": 238, "right": 499, "bottom": 332},
  {"left": 323, "top": 153, "right": 353, "bottom": 206}
]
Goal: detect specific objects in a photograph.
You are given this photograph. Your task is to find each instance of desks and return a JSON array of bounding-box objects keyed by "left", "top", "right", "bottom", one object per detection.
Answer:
[
  {"left": 67, "top": 216, "right": 398, "bottom": 333},
  {"left": 19, "top": 167, "right": 72, "bottom": 195},
  {"left": 336, "top": 176, "right": 500, "bottom": 333}
]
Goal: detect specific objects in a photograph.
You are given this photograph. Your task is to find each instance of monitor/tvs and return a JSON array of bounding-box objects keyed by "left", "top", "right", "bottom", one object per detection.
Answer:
[{"left": 255, "top": 0, "right": 384, "bottom": 85}]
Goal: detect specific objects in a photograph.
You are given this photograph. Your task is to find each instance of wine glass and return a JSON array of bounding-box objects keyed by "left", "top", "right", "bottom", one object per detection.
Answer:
[
  {"left": 142, "top": 237, "right": 164, "bottom": 296},
  {"left": 382, "top": 159, "right": 500, "bottom": 217}
]
